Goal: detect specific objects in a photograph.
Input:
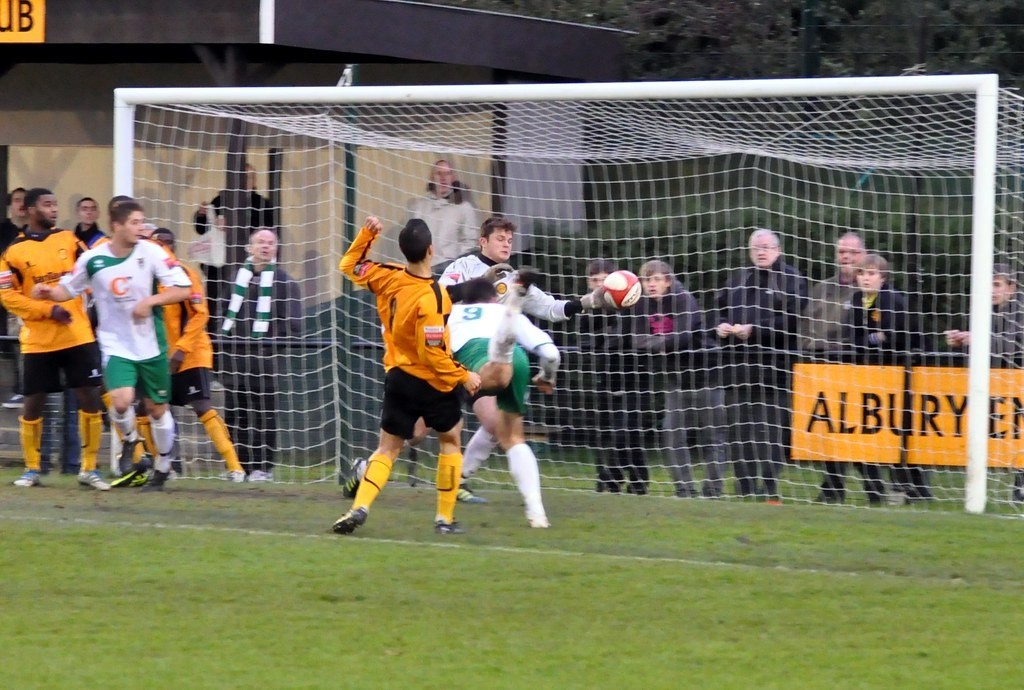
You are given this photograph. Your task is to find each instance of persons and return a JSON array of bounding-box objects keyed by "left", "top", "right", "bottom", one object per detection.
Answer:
[
  {"left": 193, "top": 162, "right": 273, "bottom": 378},
  {"left": 221, "top": 229, "right": 303, "bottom": 483},
  {"left": 0, "top": 185, "right": 223, "bottom": 480},
  {"left": 343, "top": 216, "right": 618, "bottom": 502},
  {"left": 414, "top": 160, "right": 480, "bottom": 269},
  {"left": 137, "top": 226, "right": 248, "bottom": 484},
  {"left": 802, "top": 233, "right": 937, "bottom": 505},
  {"left": 568, "top": 258, "right": 728, "bottom": 497},
  {"left": 0, "top": 187, "right": 113, "bottom": 492},
  {"left": 945, "top": 263, "right": 1024, "bottom": 500},
  {"left": 332, "top": 215, "right": 483, "bottom": 537},
  {"left": 31, "top": 199, "right": 192, "bottom": 488},
  {"left": 446, "top": 268, "right": 560, "bottom": 530},
  {"left": 713, "top": 228, "right": 809, "bottom": 504}
]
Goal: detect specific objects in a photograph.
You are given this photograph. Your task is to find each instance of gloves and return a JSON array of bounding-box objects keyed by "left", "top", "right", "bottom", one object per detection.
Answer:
[
  {"left": 579, "top": 287, "right": 605, "bottom": 310},
  {"left": 51, "top": 305, "right": 71, "bottom": 324},
  {"left": 170, "top": 351, "right": 184, "bottom": 372},
  {"left": 479, "top": 263, "right": 514, "bottom": 285}
]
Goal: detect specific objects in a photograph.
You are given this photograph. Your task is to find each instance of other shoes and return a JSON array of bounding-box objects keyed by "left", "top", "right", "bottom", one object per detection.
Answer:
[
  {"left": 231, "top": 473, "right": 247, "bottom": 482},
  {"left": 249, "top": 471, "right": 273, "bottom": 483},
  {"left": 145, "top": 471, "right": 168, "bottom": 491},
  {"left": 119, "top": 437, "right": 144, "bottom": 473}
]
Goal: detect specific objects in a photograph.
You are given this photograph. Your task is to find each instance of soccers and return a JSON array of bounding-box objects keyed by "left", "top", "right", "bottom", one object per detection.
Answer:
[{"left": 602, "top": 269, "right": 642, "bottom": 309}]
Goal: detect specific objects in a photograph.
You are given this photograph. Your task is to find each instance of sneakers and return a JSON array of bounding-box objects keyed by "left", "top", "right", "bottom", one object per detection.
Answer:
[
  {"left": 493, "top": 268, "right": 540, "bottom": 297},
  {"left": 457, "top": 484, "right": 485, "bottom": 504},
  {"left": 436, "top": 518, "right": 464, "bottom": 534},
  {"left": 325, "top": 506, "right": 369, "bottom": 537},
  {"left": 343, "top": 457, "right": 367, "bottom": 498},
  {"left": 14, "top": 470, "right": 39, "bottom": 487},
  {"left": 76, "top": 471, "right": 110, "bottom": 491},
  {"left": 526, "top": 509, "right": 552, "bottom": 529}
]
